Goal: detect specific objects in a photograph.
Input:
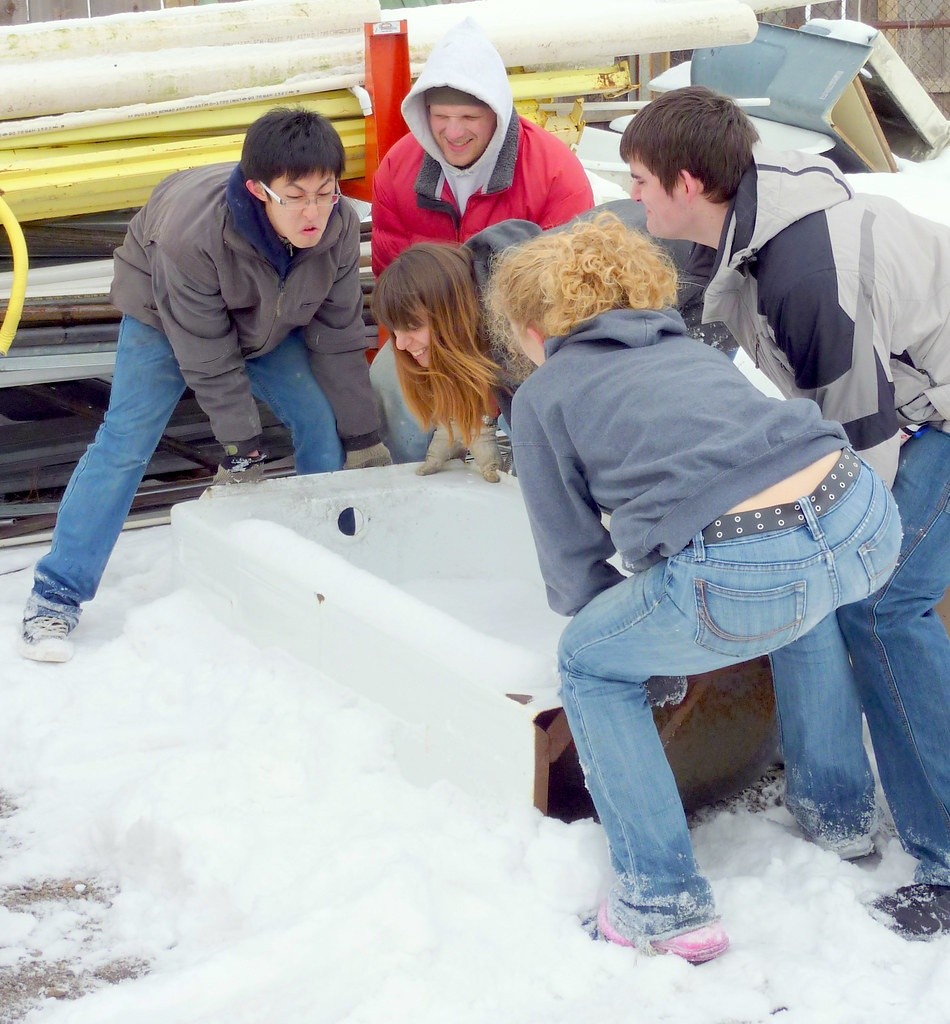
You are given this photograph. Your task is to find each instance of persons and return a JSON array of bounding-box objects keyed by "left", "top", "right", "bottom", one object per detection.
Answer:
[
  {"left": 618, "top": 86, "right": 950, "bottom": 942},
  {"left": 481, "top": 205, "right": 902, "bottom": 965},
  {"left": 371, "top": 196, "right": 697, "bottom": 482},
  {"left": 17, "top": 107, "right": 393, "bottom": 662},
  {"left": 364, "top": 26, "right": 597, "bottom": 464}
]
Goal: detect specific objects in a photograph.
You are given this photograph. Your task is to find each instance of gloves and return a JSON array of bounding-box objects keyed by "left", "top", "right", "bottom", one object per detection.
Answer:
[
  {"left": 415, "top": 421, "right": 504, "bottom": 483},
  {"left": 210, "top": 450, "right": 268, "bottom": 485},
  {"left": 342, "top": 442, "right": 393, "bottom": 470}
]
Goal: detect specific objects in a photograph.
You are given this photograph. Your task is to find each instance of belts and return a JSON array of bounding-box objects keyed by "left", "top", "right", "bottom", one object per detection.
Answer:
[{"left": 684, "top": 446, "right": 861, "bottom": 548}]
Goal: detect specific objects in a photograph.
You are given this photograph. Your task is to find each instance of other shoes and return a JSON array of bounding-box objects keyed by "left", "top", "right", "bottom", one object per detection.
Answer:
[
  {"left": 865, "top": 883, "right": 950, "bottom": 941},
  {"left": 578, "top": 897, "right": 730, "bottom": 962},
  {"left": 840, "top": 842, "right": 877, "bottom": 862}
]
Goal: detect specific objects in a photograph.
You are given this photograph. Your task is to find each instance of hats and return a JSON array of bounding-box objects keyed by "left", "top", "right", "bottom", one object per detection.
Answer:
[{"left": 425, "top": 86, "right": 487, "bottom": 109}]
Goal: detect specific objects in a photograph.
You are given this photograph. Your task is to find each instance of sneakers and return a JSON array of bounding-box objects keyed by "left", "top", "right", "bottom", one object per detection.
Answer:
[{"left": 18, "top": 598, "right": 84, "bottom": 662}]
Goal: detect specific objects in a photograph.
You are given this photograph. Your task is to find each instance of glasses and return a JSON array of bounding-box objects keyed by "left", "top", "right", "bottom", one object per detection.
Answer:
[{"left": 259, "top": 179, "right": 342, "bottom": 209}]
